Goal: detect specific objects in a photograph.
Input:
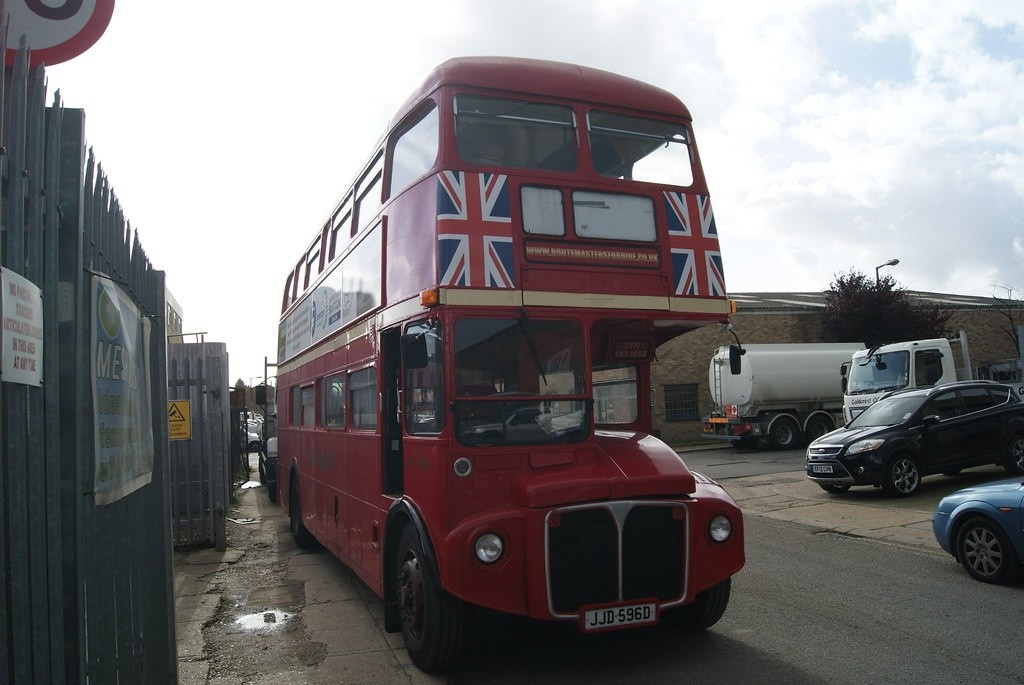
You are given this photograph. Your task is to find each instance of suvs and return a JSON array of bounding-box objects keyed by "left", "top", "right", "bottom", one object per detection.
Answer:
[{"left": 805, "top": 380, "right": 1023, "bottom": 499}]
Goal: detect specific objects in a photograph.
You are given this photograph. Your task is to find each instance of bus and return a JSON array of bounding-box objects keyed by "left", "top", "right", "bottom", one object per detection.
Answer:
[{"left": 275, "top": 55, "right": 750, "bottom": 678}]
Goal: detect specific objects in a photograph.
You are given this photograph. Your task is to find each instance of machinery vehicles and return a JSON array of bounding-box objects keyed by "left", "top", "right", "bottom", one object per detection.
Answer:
[{"left": 701, "top": 343, "right": 900, "bottom": 451}]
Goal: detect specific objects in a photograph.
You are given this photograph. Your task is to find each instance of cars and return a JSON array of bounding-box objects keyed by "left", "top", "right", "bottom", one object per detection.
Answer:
[
  {"left": 257, "top": 415, "right": 277, "bottom": 504},
  {"left": 239, "top": 411, "right": 266, "bottom": 453},
  {"left": 931, "top": 477, "right": 1024, "bottom": 583}
]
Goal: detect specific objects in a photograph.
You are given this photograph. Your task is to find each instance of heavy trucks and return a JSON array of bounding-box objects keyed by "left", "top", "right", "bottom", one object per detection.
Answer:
[{"left": 839, "top": 329, "right": 1024, "bottom": 425}]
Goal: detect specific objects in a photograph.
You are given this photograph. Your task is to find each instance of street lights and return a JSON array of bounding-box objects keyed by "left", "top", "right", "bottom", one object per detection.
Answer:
[
  {"left": 251, "top": 376, "right": 261, "bottom": 407},
  {"left": 874, "top": 258, "right": 900, "bottom": 289}
]
